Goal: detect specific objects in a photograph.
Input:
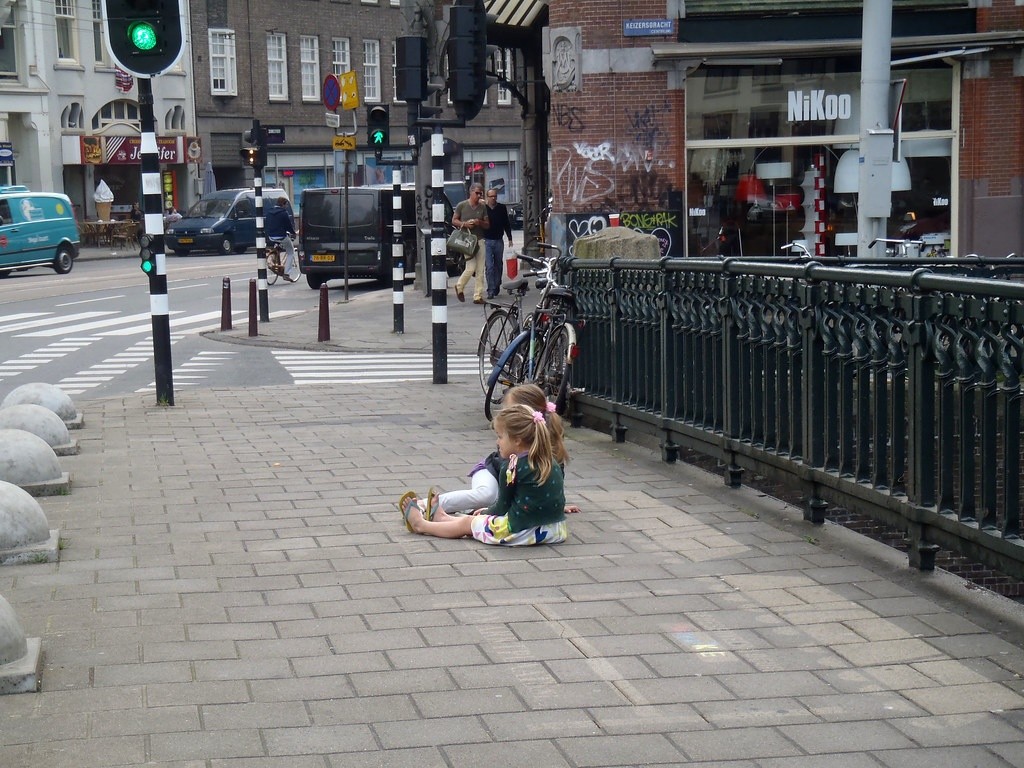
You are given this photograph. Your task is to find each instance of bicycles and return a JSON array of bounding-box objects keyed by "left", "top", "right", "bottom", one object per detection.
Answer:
[
  {"left": 264, "top": 230, "right": 302, "bottom": 285},
  {"left": 476, "top": 241, "right": 582, "bottom": 425},
  {"left": 779, "top": 238, "right": 1020, "bottom": 282}
]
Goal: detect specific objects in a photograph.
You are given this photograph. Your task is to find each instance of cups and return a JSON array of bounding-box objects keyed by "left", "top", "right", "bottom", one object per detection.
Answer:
[{"left": 609, "top": 214, "right": 620, "bottom": 227}]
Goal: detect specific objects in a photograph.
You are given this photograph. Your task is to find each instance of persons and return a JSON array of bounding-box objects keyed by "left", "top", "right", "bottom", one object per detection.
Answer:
[
  {"left": 399, "top": 403, "right": 567, "bottom": 546},
  {"left": 167, "top": 207, "right": 182, "bottom": 226},
  {"left": 484, "top": 190, "right": 513, "bottom": 298},
  {"left": 266, "top": 197, "right": 296, "bottom": 281},
  {"left": 130, "top": 201, "right": 143, "bottom": 240},
  {"left": 452, "top": 183, "right": 489, "bottom": 304},
  {"left": 418, "top": 384, "right": 581, "bottom": 513}
]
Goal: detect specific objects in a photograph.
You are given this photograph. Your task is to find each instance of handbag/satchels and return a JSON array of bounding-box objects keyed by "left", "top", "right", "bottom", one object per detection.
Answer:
[
  {"left": 506, "top": 246, "right": 518, "bottom": 279},
  {"left": 445, "top": 251, "right": 466, "bottom": 277},
  {"left": 446, "top": 221, "right": 478, "bottom": 261}
]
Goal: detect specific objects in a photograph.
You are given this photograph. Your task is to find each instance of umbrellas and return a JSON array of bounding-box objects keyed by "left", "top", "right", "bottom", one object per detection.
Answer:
[{"left": 203, "top": 162, "right": 216, "bottom": 196}]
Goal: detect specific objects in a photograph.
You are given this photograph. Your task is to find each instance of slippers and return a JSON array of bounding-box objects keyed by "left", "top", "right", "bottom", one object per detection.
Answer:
[
  {"left": 399, "top": 491, "right": 421, "bottom": 532},
  {"left": 426, "top": 487, "right": 440, "bottom": 521}
]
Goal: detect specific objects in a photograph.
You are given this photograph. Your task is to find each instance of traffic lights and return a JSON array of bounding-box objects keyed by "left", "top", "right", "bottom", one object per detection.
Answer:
[
  {"left": 416, "top": 83, "right": 447, "bottom": 151},
  {"left": 239, "top": 146, "right": 262, "bottom": 169},
  {"left": 139, "top": 233, "right": 157, "bottom": 278},
  {"left": 365, "top": 102, "right": 391, "bottom": 153},
  {"left": 242, "top": 129, "right": 253, "bottom": 143},
  {"left": 100, "top": 0, "right": 190, "bottom": 77}
]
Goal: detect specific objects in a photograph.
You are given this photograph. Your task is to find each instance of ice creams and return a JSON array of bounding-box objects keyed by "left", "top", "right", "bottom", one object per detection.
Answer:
[{"left": 93, "top": 179, "right": 114, "bottom": 221}]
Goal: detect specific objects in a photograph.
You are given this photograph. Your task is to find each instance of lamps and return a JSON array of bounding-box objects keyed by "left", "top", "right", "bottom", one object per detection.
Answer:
[
  {"left": 891, "top": 46, "right": 994, "bottom": 65},
  {"left": 755, "top": 162, "right": 792, "bottom": 256},
  {"left": 703, "top": 57, "right": 783, "bottom": 65},
  {"left": 786, "top": 178, "right": 796, "bottom": 211},
  {"left": 750, "top": 175, "right": 763, "bottom": 212}
]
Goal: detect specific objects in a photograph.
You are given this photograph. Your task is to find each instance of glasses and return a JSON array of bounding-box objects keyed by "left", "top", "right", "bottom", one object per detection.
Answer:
[
  {"left": 488, "top": 195, "right": 498, "bottom": 198},
  {"left": 475, "top": 191, "right": 483, "bottom": 196}
]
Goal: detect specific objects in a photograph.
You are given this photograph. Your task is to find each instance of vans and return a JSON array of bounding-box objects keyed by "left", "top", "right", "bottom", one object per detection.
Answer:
[
  {"left": 0, "top": 191, "right": 81, "bottom": 279},
  {"left": 366, "top": 181, "right": 467, "bottom": 216},
  {"left": 298, "top": 186, "right": 467, "bottom": 285},
  {"left": 163, "top": 186, "right": 296, "bottom": 255}
]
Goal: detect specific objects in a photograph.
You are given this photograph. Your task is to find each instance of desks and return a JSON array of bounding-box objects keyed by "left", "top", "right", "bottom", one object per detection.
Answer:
[{"left": 85, "top": 221, "right": 125, "bottom": 248}]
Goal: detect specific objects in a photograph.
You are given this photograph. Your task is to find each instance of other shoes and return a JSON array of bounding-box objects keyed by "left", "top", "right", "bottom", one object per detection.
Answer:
[
  {"left": 487, "top": 294, "right": 494, "bottom": 299},
  {"left": 474, "top": 299, "right": 485, "bottom": 304},
  {"left": 283, "top": 274, "right": 293, "bottom": 282},
  {"left": 455, "top": 285, "right": 465, "bottom": 302}
]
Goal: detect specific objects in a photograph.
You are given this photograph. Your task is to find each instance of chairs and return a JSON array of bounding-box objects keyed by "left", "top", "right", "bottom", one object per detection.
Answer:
[{"left": 77, "top": 218, "right": 140, "bottom": 251}]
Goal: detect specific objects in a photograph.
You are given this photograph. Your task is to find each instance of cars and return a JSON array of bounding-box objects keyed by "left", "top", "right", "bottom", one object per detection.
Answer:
[
  {"left": 508, "top": 190, "right": 553, "bottom": 232},
  {"left": 740, "top": 146, "right": 858, "bottom": 236}
]
